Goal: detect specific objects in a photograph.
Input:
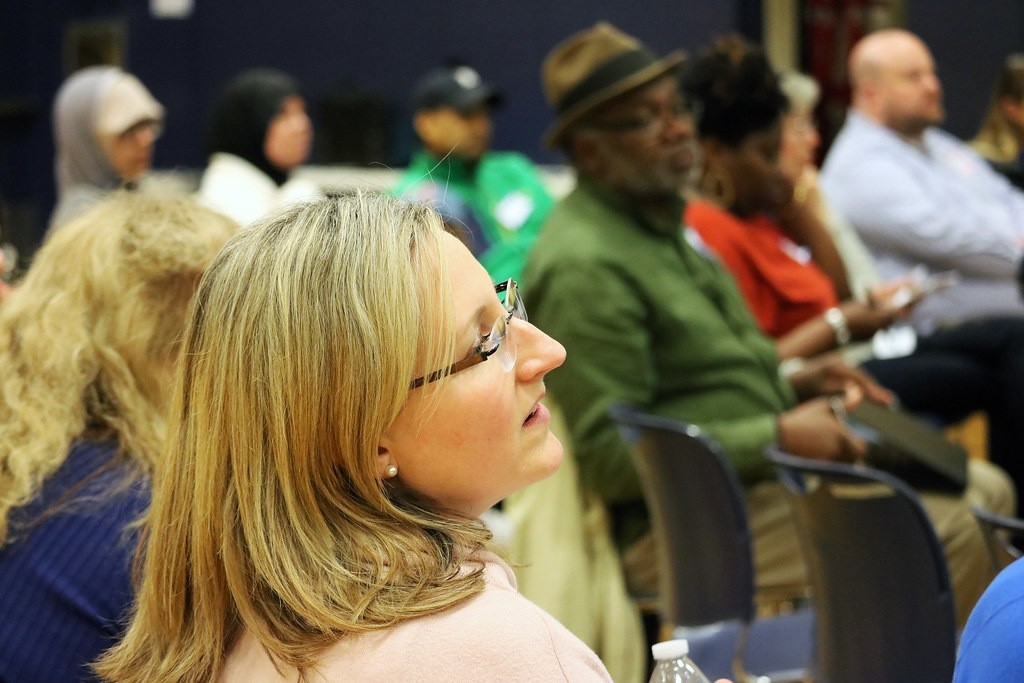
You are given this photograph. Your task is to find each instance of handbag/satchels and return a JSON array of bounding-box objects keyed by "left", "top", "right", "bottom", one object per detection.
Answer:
[{"left": 842, "top": 396, "right": 970, "bottom": 494}]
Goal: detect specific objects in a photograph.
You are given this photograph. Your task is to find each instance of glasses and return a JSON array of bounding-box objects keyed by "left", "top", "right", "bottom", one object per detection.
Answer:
[
  {"left": 589, "top": 87, "right": 693, "bottom": 133},
  {"left": 408, "top": 279, "right": 527, "bottom": 391}
]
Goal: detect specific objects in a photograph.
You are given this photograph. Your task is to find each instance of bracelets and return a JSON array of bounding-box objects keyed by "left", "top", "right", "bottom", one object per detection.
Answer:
[{"left": 824, "top": 307, "right": 850, "bottom": 345}]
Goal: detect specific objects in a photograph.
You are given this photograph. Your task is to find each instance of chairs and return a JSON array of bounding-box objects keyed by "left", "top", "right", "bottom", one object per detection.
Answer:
[
  {"left": 608, "top": 402, "right": 814, "bottom": 683},
  {"left": 967, "top": 507, "right": 1024, "bottom": 572},
  {"left": 760, "top": 446, "right": 956, "bottom": 683}
]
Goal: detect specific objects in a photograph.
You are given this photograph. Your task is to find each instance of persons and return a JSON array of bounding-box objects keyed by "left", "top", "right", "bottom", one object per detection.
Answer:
[
  {"left": 676, "top": 34, "right": 1024, "bottom": 551},
  {"left": 92, "top": 190, "right": 613, "bottom": 683},
  {"left": 199, "top": 68, "right": 312, "bottom": 228},
  {"left": 515, "top": 21, "right": 1015, "bottom": 641},
  {"left": 0, "top": 196, "right": 251, "bottom": 683},
  {"left": 396, "top": 65, "right": 554, "bottom": 299},
  {"left": 48, "top": 64, "right": 166, "bottom": 232}
]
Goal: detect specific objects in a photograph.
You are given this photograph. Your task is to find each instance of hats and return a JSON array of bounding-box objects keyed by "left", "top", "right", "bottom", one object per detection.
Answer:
[
  {"left": 412, "top": 65, "right": 506, "bottom": 119},
  {"left": 538, "top": 22, "right": 690, "bottom": 149},
  {"left": 89, "top": 70, "right": 165, "bottom": 137}
]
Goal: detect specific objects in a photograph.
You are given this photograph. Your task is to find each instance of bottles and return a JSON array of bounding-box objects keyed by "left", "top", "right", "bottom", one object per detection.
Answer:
[{"left": 648, "top": 639, "right": 709, "bottom": 683}]
